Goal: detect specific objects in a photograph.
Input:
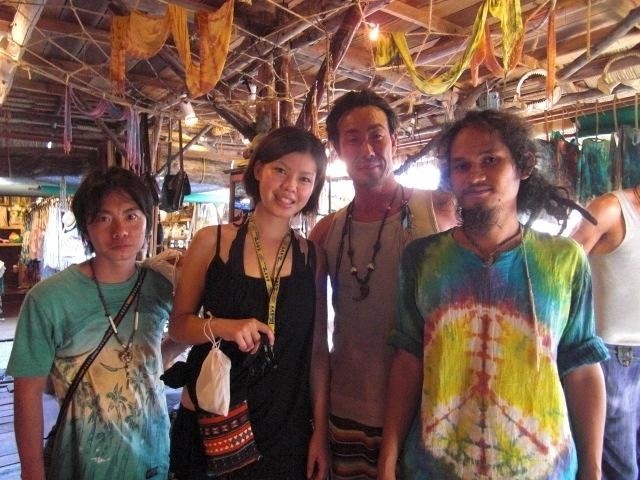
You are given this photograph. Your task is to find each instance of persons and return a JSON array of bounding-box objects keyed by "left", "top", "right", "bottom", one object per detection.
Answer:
[
  {"left": 168, "top": 127, "right": 331, "bottom": 479},
  {"left": 379, "top": 109, "right": 606, "bottom": 479},
  {"left": 307, "top": 88, "right": 458, "bottom": 478},
  {"left": 6, "top": 166, "right": 190, "bottom": 478},
  {"left": 568, "top": 182, "right": 640, "bottom": 480}
]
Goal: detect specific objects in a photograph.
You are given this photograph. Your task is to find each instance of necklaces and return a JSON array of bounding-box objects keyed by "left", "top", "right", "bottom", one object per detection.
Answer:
[
  {"left": 89, "top": 258, "right": 141, "bottom": 389},
  {"left": 334, "top": 185, "right": 412, "bottom": 301},
  {"left": 633, "top": 188, "right": 640, "bottom": 205},
  {"left": 461, "top": 229, "right": 522, "bottom": 267}
]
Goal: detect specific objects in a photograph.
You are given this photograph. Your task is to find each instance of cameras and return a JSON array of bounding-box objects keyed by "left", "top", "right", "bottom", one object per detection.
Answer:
[{"left": 230, "top": 329, "right": 281, "bottom": 385}]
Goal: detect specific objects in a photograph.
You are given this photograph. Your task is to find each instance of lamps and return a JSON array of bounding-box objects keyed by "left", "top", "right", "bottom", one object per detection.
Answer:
[
  {"left": 368, "top": 23, "right": 381, "bottom": 39},
  {"left": 180, "top": 100, "right": 199, "bottom": 126}
]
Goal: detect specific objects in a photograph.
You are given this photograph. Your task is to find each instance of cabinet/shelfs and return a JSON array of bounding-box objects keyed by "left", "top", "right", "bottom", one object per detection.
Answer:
[
  {"left": 222, "top": 166, "right": 256, "bottom": 222},
  {"left": 0, "top": 195, "right": 25, "bottom": 292},
  {"left": 156, "top": 206, "right": 194, "bottom": 249}
]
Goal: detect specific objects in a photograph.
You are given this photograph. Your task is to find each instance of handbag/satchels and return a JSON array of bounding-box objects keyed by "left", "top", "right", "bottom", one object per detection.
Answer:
[
  {"left": 159, "top": 170, "right": 192, "bottom": 212},
  {"left": 180, "top": 364, "right": 264, "bottom": 475}
]
